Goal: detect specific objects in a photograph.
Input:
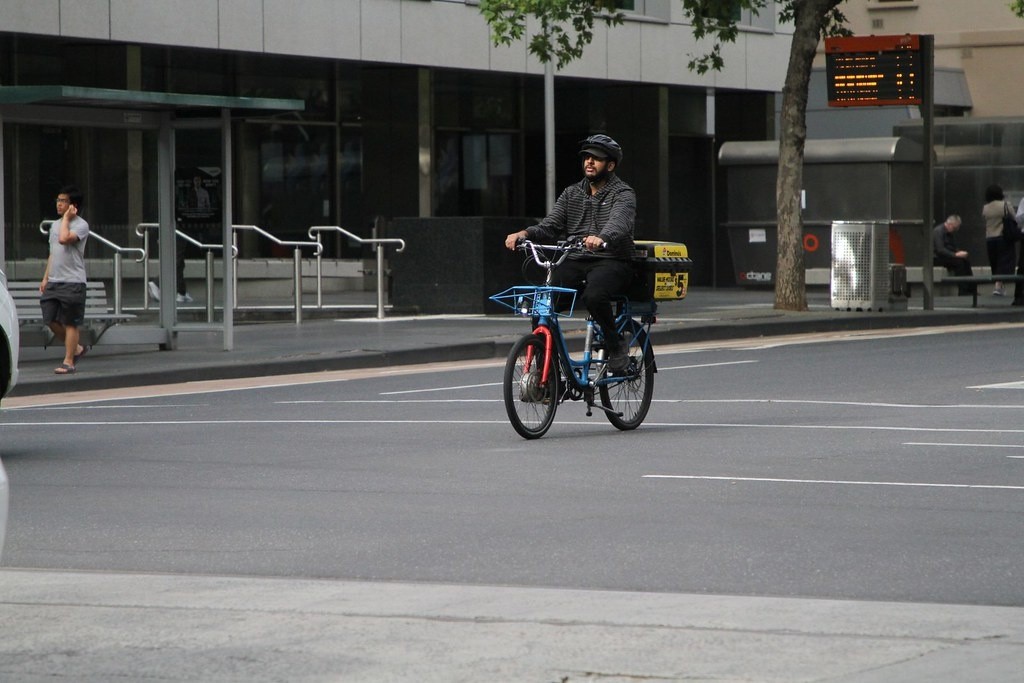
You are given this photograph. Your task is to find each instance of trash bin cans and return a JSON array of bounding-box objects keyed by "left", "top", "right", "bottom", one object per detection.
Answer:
[{"left": 828, "top": 220, "right": 890, "bottom": 312}]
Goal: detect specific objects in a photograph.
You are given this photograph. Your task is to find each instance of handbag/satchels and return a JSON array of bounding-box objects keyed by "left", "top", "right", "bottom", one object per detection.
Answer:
[{"left": 1002, "top": 214, "right": 1023, "bottom": 242}]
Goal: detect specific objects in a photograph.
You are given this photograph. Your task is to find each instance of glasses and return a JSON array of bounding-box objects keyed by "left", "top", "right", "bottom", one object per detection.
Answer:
[
  {"left": 56, "top": 198, "right": 72, "bottom": 204},
  {"left": 583, "top": 153, "right": 610, "bottom": 162}
]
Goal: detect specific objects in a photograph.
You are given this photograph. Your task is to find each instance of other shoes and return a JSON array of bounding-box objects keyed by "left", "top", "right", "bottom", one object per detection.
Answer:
[{"left": 956, "top": 289, "right": 982, "bottom": 297}]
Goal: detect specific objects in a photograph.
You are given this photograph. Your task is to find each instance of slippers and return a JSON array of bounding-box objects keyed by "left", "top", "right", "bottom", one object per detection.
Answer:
[
  {"left": 55, "top": 364, "right": 77, "bottom": 375},
  {"left": 73, "top": 345, "right": 90, "bottom": 364}
]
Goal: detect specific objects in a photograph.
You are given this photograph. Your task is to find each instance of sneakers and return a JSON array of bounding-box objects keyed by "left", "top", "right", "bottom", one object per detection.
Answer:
[
  {"left": 993, "top": 287, "right": 1010, "bottom": 296},
  {"left": 608, "top": 339, "right": 631, "bottom": 371}
]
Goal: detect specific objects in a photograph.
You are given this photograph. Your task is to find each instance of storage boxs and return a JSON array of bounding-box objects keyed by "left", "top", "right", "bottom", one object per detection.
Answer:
[{"left": 605, "top": 239, "right": 695, "bottom": 300}]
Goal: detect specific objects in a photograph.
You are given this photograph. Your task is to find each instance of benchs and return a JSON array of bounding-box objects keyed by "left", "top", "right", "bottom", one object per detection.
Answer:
[
  {"left": 902, "top": 266, "right": 996, "bottom": 299},
  {"left": 7, "top": 281, "right": 138, "bottom": 351},
  {"left": 940, "top": 274, "right": 1024, "bottom": 308}
]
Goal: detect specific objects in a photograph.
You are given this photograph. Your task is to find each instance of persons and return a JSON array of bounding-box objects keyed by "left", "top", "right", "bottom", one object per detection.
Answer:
[
  {"left": 931, "top": 214, "right": 980, "bottom": 297},
  {"left": 983, "top": 185, "right": 1019, "bottom": 296},
  {"left": 39, "top": 187, "right": 91, "bottom": 375},
  {"left": 193, "top": 175, "right": 210, "bottom": 209},
  {"left": 1010, "top": 196, "right": 1024, "bottom": 306},
  {"left": 143, "top": 186, "right": 194, "bottom": 303},
  {"left": 503, "top": 134, "right": 644, "bottom": 403}
]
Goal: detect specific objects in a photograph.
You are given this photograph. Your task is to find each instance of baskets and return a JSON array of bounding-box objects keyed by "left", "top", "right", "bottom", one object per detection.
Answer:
[{"left": 488, "top": 285, "right": 577, "bottom": 318}]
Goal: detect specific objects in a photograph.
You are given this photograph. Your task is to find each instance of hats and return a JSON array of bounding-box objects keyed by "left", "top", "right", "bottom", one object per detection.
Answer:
[{"left": 578, "top": 134, "right": 624, "bottom": 166}]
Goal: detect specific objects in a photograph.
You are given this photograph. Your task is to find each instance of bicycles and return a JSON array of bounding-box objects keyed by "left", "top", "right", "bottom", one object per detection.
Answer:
[{"left": 486, "top": 235, "right": 696, "bottom": 440}]
